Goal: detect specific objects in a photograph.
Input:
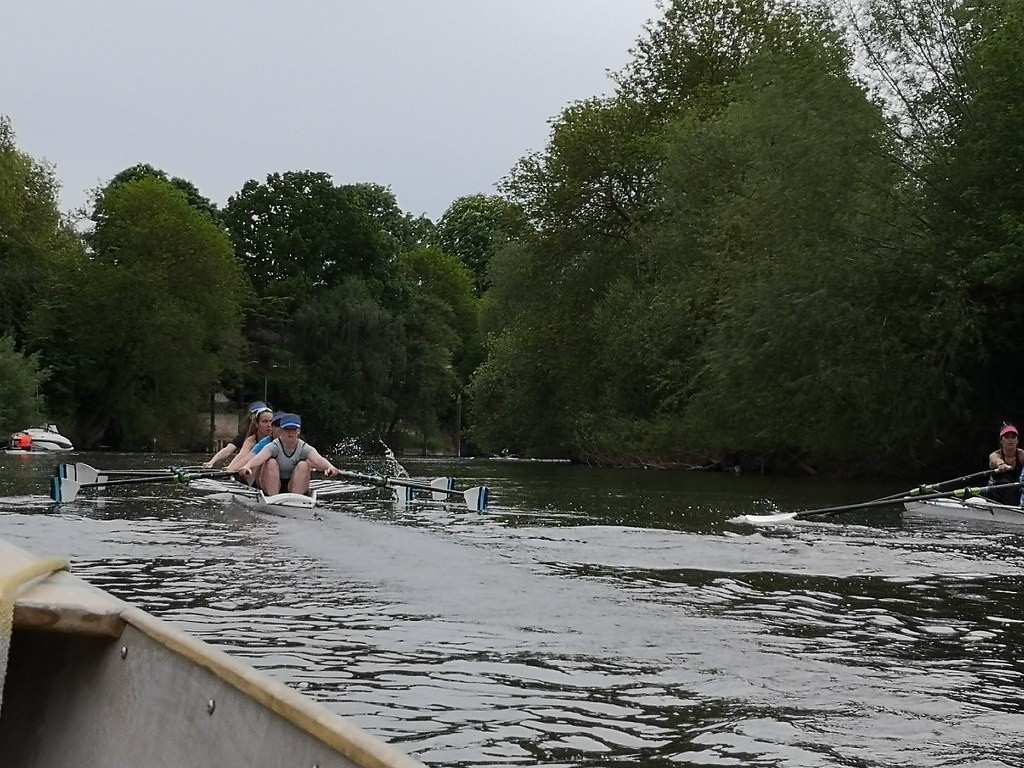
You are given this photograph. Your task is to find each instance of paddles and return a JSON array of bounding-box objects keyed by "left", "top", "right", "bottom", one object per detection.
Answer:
[
  {"left": 329, "top": 475, "right": 416, "bottom": 500},
  {"left": 328, "top": 470, "right": 490, "bottom": 511},
  {"left": 0, "top": 443, "right": 20, "bottom": 450},
  {"left": 820, "top": 469, "right": 1007, "bottom": 517},
  {"left": 56, "top": 462, "right": 223, "bottom": 489},
  {"left": 745, "top": 481, "right": 1024, "bottom": 523},
  {"left": 50, "top": 470, "right": 251, "bottom": 503},
  {"left": 81, "top": 466, "right": 204, "bottom": 490},
  {"left": 313, "top": 471, "right": 456, "bottom": 500}
]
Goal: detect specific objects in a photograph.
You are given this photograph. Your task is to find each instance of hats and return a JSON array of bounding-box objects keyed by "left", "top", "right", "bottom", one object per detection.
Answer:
[
  {"left": 270, "top": 415, "right": 285, "bottom": 424},
  {"left": 250, "top": 402, "right": 267, "bottom": 413},
  {"left": 280, "top": 419, "right": 301, "bottom": 429}
]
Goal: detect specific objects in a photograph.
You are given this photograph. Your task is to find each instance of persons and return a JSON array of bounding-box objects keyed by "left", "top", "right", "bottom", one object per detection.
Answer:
[
  {"left": 985, "top": 425, "right": 1024, "bottom": 507},
  {"left": 203, "top": 401, "right": 339, "bottom": 496},
  {"left": 20, "top": 432, "right": 31, "bottom": 451}
]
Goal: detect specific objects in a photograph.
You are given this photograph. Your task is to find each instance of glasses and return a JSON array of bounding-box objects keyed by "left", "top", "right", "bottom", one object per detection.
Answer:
[{"left": 274, "top": 421, "right": 280, "bottom": 427}]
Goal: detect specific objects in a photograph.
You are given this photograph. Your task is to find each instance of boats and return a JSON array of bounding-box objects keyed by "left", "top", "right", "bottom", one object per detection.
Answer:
[
  {"left": 8, "top": 427, "right": 74, "bottom": 452},
  {"left": 229, "top": 477, "right": 319, "bottom": 520},
  {"left": 903, "top": 495, "right": 1024, "bottom": 530}
]
